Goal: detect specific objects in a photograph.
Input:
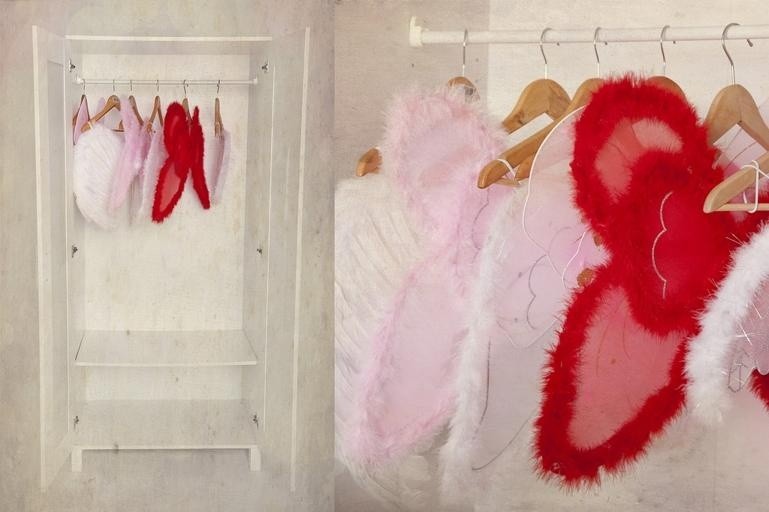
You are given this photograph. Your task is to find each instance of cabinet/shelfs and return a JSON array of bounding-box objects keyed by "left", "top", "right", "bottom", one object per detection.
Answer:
[{"left": 32, "top": 28, "right": 276, "bottom": 493}]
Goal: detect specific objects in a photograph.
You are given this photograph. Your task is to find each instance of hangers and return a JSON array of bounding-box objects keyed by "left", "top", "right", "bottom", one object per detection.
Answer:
[
  {"left": 356, "top": 21, "right": 769, "bottom": 216},
  {"left": 71, "top": 78, "right": 231, "bottom": 140}
]
